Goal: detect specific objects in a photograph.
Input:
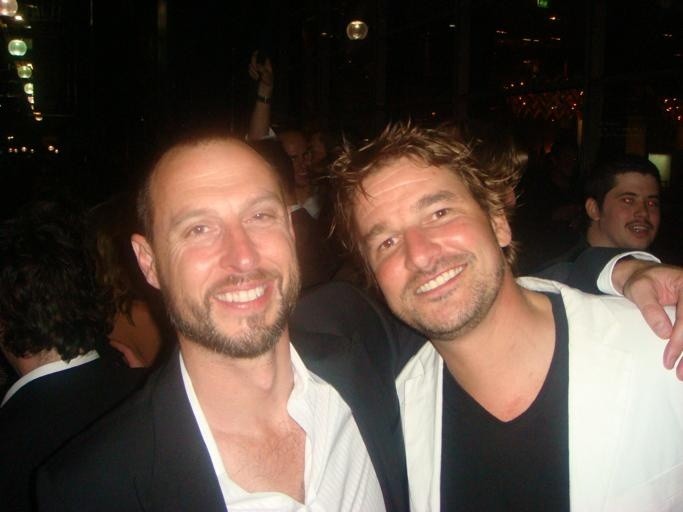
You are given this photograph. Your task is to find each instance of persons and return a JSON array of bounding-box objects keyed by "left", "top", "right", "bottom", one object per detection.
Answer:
[{"left": 0, "top": 52, "right": 683, "bottom": 512}]
